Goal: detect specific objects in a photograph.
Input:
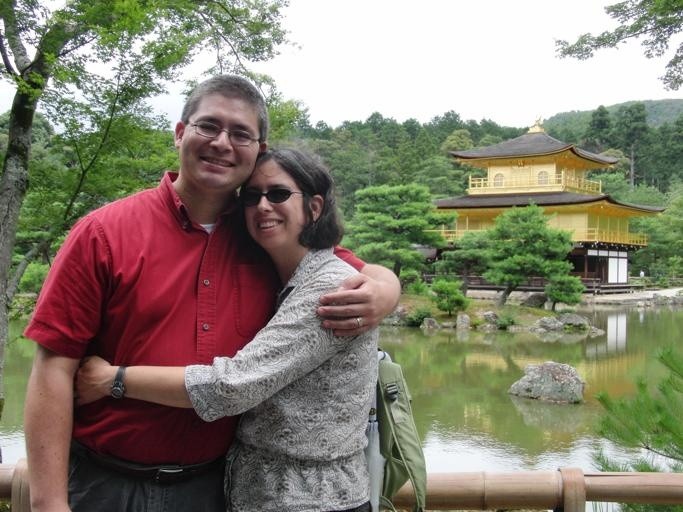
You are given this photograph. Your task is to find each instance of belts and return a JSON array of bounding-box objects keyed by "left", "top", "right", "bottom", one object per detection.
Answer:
[{"left": 68, "top": 437, "right": 227, "bottom": 487}]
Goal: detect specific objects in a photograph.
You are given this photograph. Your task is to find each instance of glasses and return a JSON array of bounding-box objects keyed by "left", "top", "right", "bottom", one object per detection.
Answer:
[
  {"left": 183, "top": 118, "right": 261, "bottom": 148},
  {"left": 238, "top": 186, "right": 304, "bottom": 207}
]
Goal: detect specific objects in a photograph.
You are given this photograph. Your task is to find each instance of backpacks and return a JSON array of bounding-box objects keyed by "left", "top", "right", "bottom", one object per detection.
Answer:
[{"left": 362, "top": 341, "right": 434, "bottom": 512}]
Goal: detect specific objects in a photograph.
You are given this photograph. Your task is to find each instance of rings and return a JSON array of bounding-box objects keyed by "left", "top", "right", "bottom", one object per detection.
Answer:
[{"left": 353, "top": 316, "right": 363, "bottom": 328}]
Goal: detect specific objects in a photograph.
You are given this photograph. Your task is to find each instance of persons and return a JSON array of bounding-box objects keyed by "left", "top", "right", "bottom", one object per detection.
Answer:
[
  {"left": 74, "top": 142, "right": 381, "bottom": 511},
  {"left": 22, "top": 73, "right": 403, "bottom": 512}
]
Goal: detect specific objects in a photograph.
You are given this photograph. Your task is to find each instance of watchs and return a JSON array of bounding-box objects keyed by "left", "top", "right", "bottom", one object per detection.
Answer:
[{"left": 109, "top": 365, "right": 126, "bottom": 402}]
